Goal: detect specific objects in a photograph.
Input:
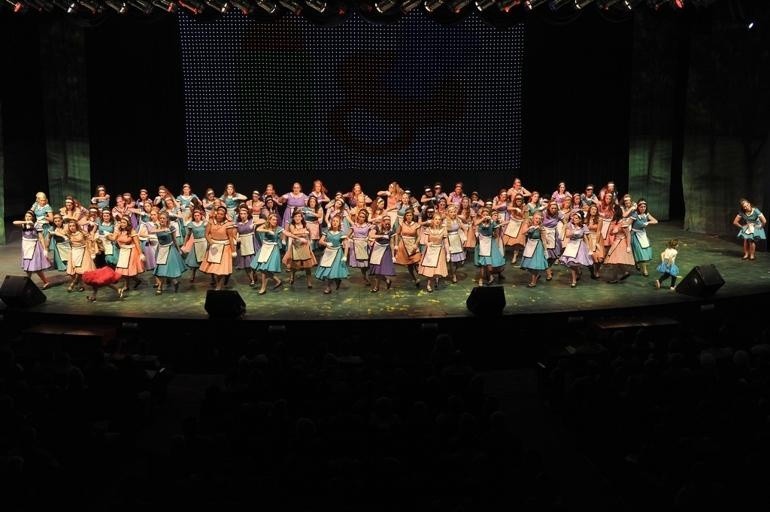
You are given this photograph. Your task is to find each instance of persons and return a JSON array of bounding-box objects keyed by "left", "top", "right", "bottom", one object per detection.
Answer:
[
  {"left": 655, "top": 238, "right": 680, "bottom": 292},
  {"left": 733, "top": 198, "right": 767, "bottom": 260},
  {"left": 13, "top": 177, "right": 660, "bottom": 303}
]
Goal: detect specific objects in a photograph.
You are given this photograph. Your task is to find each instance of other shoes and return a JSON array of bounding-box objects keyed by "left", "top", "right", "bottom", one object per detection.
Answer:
[
  {"left": 413, "top": 273, "right": 458, "bottom": 292},
  {"left": 473, "top": 268, "right": 649, "bottom": 287},
  {"left": 386, "top": 279, "right": 392, "bottom": 290},
  {"left": 307, "top": 281, "right": 312, "bottom": 289},
  {"left": 671, "top": 286, "right": 675, "bottom": 290},
  {"left": 324, "top": 289, "right": 332, "bottom": 294},
  {"left": 42, "top": 282, "right": 50, "bottom": 289},
  {"left": 67, "top": 277, "right": 282, "bottom": 301},
  {"left": 655, "top": 280, "right": 660, "bottom": 289},
  {"left": 369, "top": 287, "right": 380, "bottom": 292},
  {"left": 336, "top": 279, "right": 342, "bottom": 291},
  {"left": 290, "top": 278, "right": 294, "bottom": 284}
]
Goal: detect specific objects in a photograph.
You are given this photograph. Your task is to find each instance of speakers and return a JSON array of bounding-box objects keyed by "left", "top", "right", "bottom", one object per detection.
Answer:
[
  {"left": 205, "top": 290, "right": 245, "bottom": 318},
  {"left": 466, "top": 286, "right": 506, "bottom": 312},
  {"left": 0, "top": 275, "right": 46, "bottom": 309},
  {"left": 675, "top": 264, "right": 725, "bottom": 296}
]
